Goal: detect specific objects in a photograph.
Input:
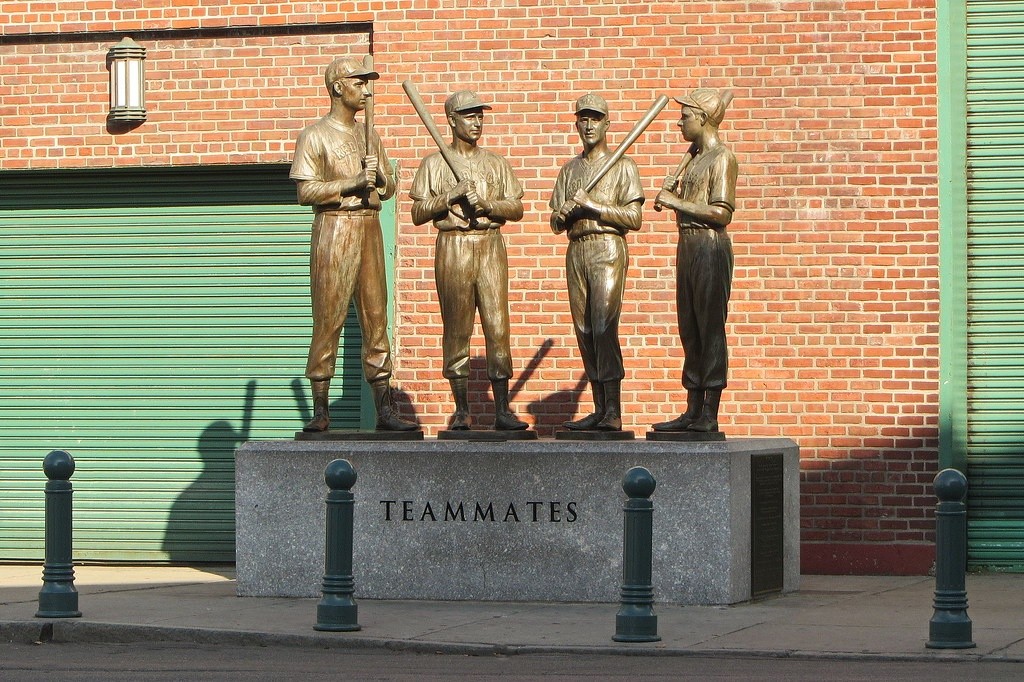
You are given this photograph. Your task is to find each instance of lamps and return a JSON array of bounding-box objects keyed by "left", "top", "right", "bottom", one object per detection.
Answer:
[{"left": 107, "top": 37, "right": 146, "bottom": 122}]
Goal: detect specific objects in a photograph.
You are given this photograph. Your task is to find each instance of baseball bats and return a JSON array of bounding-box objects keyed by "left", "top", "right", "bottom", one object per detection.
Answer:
[
  {"left": 653, "top": 91, "right": 736, "bottom": 213},
  {"left": 402, "top": 78, "right": 485, "bottom": 217},
  {"left": 556, "top": 94, "right": 670, "bottom": 223},
  {"left": 361, "top": 54, "right": 376, "bottom": 192}
]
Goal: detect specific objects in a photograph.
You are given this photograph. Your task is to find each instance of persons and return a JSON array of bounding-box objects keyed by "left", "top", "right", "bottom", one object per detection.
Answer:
[
  {"left": 289, "top": 58, "right": 420, "bottom": 434},
  {"left": 546, "top": 96, "right": 645, "bottom": 429},
  {"left": 410, "top": 90, "right": 531, "bottom": 432},
  {"left": 655, "top": 90, "right": 739, "bottom": 434}
]
